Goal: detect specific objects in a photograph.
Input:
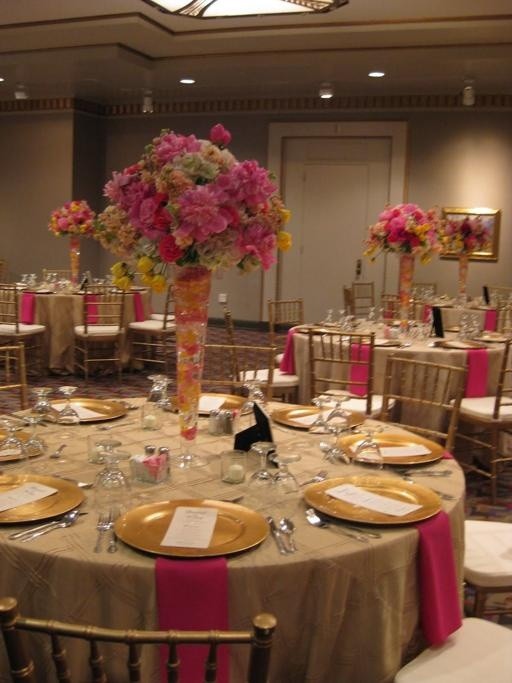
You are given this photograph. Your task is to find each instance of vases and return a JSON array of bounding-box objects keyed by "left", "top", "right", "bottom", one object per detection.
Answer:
[
  {"left": 169, "top": 264, "right": 221, "bottom": 486},
  {"left": 394, "top": 253, "right": 417, "bottom": 342},
  {"left": 455, "top": 257, "right": 469, "bottom": 305},
  {"left": 67, "top": 234, "right": 82, "bottom": 289}
]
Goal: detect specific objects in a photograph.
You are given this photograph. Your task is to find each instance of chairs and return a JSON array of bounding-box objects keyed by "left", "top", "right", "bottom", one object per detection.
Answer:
[
  {"left": 1, "top": 269, "right": 510, "bottom": 400},
  {"left": 447, "top": 341, "right": 511, "bottom": 503},
  {"left": 307, "top": 326, "right": 375, "bottom": 417},
  {"left": 393, "top": 618, "right": 510, "bottom": 682},
  {"left": 2, "top": 597, "right": 275, "bottom": 682},
  {"left": 1, "top": 343, "right": 25, "bottom": 415},
  {"left": 380, "top": 352, "right": 469, "bottom": 455},
  {"left": 461, "top": 521, "right": 512, "bottom": 619}
]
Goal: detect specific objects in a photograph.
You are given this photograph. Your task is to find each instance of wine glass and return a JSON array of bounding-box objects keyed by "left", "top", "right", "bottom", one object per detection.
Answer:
[
  {"left": 16, "top": 268, "right": 148, "bottom": 295},
  {"left": 324, "top": 285, "right": 511, "bottom": 347}
]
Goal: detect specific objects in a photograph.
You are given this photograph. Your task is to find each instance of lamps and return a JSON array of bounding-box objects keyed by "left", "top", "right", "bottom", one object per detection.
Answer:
[
  {"left": 368, "top": 71, "right": 386, "bottom": 78},
  {"left": 179, "top": 79, "right": 197, "bottom": 85},
  {"left": 462, "top": 86, "right": 475, "bottom": 105},
  {"left": 318, "top": 87, "right": 334, "bottom": 99},
  {"left": 141, "top": 94, "right": 154, "bottom": 114},
  {"left": 142, "top": 0, "right": 351, "bottom": 19}
]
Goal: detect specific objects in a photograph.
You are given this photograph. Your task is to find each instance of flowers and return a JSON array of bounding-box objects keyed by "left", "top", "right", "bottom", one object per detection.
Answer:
[
  {"left": 91, "top": 123, "right": 295, "bottom": 441},
  {"left": 361, "top": 203, "right": 450, "bottom": 331},
  {"left": 46, "top": 198, "right": 99, "bottom": 282},
  {"left": 439, "top": 212, "right": 493, "bottom": 294}
]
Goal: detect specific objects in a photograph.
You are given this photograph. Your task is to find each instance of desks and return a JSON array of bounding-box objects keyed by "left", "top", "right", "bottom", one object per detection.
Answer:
[{"left": 1, "top": 394, "right": 467, "bottom": 682}]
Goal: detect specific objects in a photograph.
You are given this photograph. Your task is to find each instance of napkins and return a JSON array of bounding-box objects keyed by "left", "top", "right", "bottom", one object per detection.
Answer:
[
  {"left": 403, "top": 512, "right": 464, "bottom": 648},
  {"left": 280, "top": 330, "right": 300, "bottom": 374},
  {"left": 156, "top": 556, "right": 230, "bottom": 680},
  {"left": 350, "top": 343, "right": 370, "bottom": 394},
  {"left": 465, "top": 347, "right": 488, "bottom": 397}
]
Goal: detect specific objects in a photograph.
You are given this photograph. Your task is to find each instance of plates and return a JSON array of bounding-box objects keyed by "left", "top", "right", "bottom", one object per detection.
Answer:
[{"left": 0, "top": 373, "right": 447, "bottom": 560}]
